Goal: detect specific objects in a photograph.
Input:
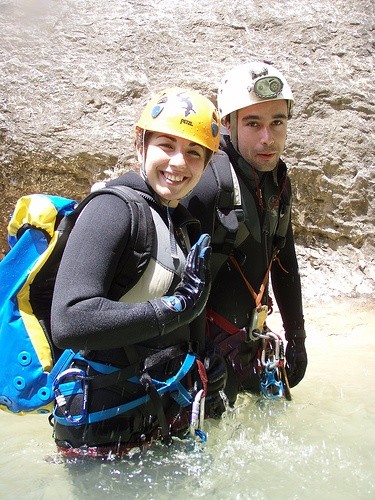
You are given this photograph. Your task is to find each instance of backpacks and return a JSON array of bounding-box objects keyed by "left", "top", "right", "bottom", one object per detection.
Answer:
[{"left": 1, "top": 185, "right": 155, "bottom": 415}]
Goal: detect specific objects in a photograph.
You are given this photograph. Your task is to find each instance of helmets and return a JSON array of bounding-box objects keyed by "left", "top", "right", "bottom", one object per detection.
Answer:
[
  {"left": 138, "top": 86, "right": 221, "bottom": 154},
  {"left": 216, "top": 61, "right": 296, "bottom": 121}
]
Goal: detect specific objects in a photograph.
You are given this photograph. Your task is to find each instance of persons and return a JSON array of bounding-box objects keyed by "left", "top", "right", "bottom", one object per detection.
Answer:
[
  {"left": 50, "top": 86, "right": 220, "bottom": 451},
  {"left": 178, "top": 62, "right": 308, "bottom": 419}
]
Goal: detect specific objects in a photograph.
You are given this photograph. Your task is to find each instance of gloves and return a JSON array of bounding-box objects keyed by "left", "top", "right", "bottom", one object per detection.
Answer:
[
  {"left": 147, "top": 233, "right": 212, "bottom": 338},
  {"left": 284, "top": 330, "right": 308, "bottom": 388}
]
